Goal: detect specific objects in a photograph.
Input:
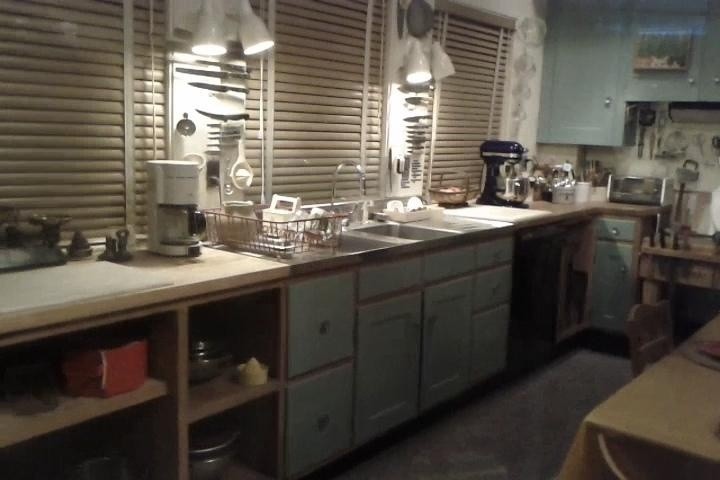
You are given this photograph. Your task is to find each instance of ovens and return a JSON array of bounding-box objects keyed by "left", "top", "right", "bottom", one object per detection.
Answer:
[{"left": 509, "top": 218, "right": 569, "bottom": 370}]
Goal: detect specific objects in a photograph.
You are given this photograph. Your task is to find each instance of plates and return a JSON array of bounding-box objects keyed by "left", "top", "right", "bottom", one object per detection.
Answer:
[{"left": 405, "top": 0, "right": 434, "bottom": 38}]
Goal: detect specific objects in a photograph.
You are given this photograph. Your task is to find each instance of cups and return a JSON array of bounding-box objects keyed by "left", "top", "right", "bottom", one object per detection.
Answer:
[
  {"left": 574, "top": 181, "right": 589, "bottom": 204},
  {"left": 387, "top": 197, "right": 425, "bottom": 214}
]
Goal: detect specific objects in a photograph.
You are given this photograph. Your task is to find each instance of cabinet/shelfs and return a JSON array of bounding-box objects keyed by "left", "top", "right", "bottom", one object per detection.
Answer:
[
  {"left": 281, "top": 268, "right": 354, "bottom": 480},
  {"left": 583, "top": 213, "right": 675, "bottom": 336},
  {"left": 0, "top": 285, "right": 281, "bottom": 479},
  {"left": 623, "top": 8, "right": 720, "bottom": 105},
  {"left": 537, "top": 8, "right": 640, "bottom": 148},
  {"left": 355, "top": 255, "right": 421, "bottom": 452},
  {"left": 506, "top": 222, "right": 595, "bottom": 376},
  {"left": 421, "top": 239, "right": 513, "bottom": 416}
]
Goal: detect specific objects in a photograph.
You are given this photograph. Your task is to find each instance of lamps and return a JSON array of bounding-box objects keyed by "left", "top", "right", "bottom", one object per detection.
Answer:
[
  {"left": 401, "top": 35, "right": 457, "bottom": 87},
  {"left": 190, "top": 0, "right": 277, "bottom": 60}
]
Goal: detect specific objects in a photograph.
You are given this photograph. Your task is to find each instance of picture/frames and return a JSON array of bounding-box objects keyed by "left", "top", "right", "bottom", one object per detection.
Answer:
[{"left": 630, "top": 26, "right": 693, "bottom": 73}]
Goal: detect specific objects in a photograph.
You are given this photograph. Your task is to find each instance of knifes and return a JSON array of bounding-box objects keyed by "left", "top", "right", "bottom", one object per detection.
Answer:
[
  {"left": 175, "top": 67, "right": 250, "bottom": 81},
  {"left": 398, "top": 85, "right": 433, "bottom": 188},
  {"left": 194, "top": 58, "right": 249, "bottom": 72},
  {"left": 194, "top": 107, "right": 250, "bottom": 121},
  {"left": 187, "top": 82, "right": 249, "bottom": 95}
]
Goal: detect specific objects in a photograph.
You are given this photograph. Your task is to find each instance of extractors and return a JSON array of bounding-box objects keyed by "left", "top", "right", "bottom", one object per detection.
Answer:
[{"left": 668, "top": 102, "right": 719, "bottom": 123}]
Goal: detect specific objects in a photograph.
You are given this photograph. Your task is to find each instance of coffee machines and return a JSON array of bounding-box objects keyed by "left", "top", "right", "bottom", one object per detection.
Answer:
[{"left": 144, "top": 158, "right": 206, "bottom": 256}]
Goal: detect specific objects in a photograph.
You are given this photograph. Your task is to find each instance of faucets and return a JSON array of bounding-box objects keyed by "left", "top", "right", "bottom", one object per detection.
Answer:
[{"left": 330, "top": 159, "right": 369, "bottom": 224}]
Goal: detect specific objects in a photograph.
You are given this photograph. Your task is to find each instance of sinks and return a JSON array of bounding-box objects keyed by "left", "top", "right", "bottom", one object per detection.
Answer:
[{"left": 340, "top": 222, "right": 460, "bottom": 241}]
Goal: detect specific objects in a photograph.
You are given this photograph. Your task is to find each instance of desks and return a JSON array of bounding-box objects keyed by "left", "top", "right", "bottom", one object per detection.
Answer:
[
  {"left": 638, "top": 234, "right": 720, "bottom": 346},
  {"left": 580, "top": 309, "right": 720, "bottom": 478}
]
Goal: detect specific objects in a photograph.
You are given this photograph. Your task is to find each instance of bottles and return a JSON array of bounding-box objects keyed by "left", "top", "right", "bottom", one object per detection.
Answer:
[{"left": 216, "top": 200, "right": 259, "bottom": 249}]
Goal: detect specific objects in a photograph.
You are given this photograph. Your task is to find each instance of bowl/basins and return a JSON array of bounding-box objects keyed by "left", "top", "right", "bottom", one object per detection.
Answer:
[
  {"left": 552, "top": 186, "right": 573, "bottom": 203},
  {"left": 188, "top": 328, "right": 233, "bottom": 381},
  {"left": 189, "top": 421, "right": 243, "bottom": 478}
]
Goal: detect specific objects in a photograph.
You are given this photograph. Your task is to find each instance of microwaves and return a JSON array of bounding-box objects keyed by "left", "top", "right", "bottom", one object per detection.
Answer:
[{"left": 605, "top": 172, "right": 673, "bottom": 207}]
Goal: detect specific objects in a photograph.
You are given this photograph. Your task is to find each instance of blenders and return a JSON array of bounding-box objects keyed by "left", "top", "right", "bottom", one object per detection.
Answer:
[{"left": 475, "top": 138, "right": 535, "bottom": 211}]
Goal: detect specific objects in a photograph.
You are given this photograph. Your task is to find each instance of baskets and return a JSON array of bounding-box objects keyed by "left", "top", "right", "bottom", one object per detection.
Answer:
[{"left": 429, "top": 171, "right": 480, "bottom": 205}]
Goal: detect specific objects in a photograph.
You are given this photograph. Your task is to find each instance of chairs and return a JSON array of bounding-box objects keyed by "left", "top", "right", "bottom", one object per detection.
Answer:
[{"left": 621, "top": 300, "right": 677, "bottom": 379}]
